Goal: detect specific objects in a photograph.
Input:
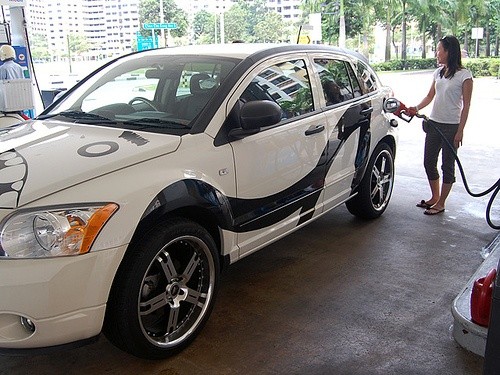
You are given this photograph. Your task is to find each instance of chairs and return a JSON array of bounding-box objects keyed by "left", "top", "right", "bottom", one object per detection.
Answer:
[{"left": 173, "top": 74, "right": 212, "bottom": 116}]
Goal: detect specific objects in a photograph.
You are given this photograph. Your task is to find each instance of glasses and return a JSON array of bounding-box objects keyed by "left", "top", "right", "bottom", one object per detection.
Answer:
[{"left": 441, "top": 35, "right": 451, "bottom": 47}]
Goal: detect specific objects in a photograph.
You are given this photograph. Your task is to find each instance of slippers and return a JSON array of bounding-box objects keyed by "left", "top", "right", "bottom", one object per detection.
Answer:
[
  {"left": 415, "top": 200, "right": 433, "bottom": 207},
  {"left": 423, "top": 205, "right": 446, "bottom": 215}
]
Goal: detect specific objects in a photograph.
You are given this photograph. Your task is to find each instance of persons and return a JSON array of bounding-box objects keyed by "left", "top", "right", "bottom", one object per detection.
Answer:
[
  {"left": 0, "top": 45, "right": 25, "bottom": 116},
  {"left": 405, "top": 35, "right": 473, "bottom": 215}
]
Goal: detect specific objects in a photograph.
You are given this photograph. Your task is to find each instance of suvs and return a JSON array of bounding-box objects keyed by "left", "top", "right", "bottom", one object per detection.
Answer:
[{"left": 0, "top": 42, "right": 398, "bottom": 363}]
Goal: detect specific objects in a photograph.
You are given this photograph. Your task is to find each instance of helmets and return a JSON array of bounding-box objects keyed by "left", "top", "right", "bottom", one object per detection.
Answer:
[{"left": 0, "top": 44, "right": 17, "bottom": 62}]
